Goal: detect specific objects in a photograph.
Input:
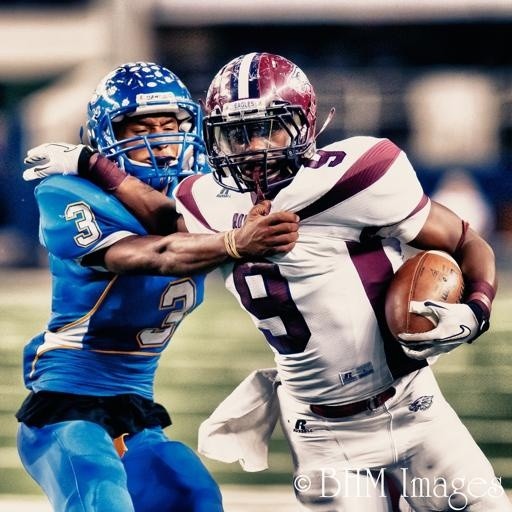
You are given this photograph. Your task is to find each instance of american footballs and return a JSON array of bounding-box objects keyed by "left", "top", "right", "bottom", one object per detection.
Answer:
[{"left": 385, "top": 249, "right": 465, "bottom": 340}]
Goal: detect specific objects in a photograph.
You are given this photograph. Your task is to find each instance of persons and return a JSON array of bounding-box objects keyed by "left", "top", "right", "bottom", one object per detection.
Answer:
[
  {"left": 21, "top": 51, "right": 512, "bottom": 512},
  {"left": 13, "top": 59, "right": 300, "bottom": 512}
]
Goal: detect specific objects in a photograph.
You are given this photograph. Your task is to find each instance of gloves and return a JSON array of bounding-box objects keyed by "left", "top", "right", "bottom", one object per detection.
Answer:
[{"left": 397, "top": 297, "right": 489, "bottom": 360}]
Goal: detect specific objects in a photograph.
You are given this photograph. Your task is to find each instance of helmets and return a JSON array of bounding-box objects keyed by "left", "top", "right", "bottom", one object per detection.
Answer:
[
  {"left": 86, "top": 62, "right": 202, "bottom": 179},
  {"left": 202, "top": 51, "right": 318, "bottom": 191}
]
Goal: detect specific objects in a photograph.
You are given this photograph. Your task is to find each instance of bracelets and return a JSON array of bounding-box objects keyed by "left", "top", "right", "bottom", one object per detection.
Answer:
[
  {"left": 469, "top": 280, "right": 495, "bottom": 316},
  {"left": 89, "top": 152, "right": 128, "bottom": 195},
  {"left": 224, "top": 229, "right": 244, "bottom": 261}
]
